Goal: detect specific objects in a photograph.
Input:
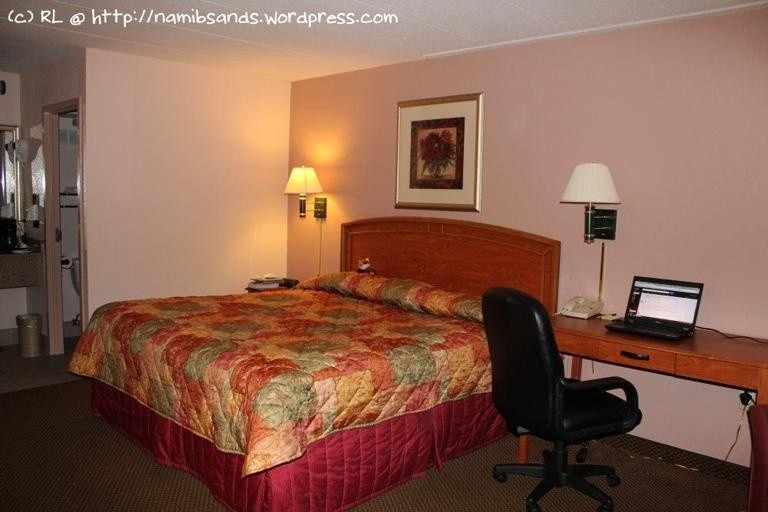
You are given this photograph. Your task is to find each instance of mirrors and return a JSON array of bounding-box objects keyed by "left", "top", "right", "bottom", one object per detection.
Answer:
[{"left": 0, "top": 125, "right": 22, "bottom": 251}]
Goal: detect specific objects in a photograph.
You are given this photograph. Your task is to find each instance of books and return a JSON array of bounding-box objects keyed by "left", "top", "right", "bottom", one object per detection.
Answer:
[{"left": 247, "top": 275, "right": 286, "bottom": 290}]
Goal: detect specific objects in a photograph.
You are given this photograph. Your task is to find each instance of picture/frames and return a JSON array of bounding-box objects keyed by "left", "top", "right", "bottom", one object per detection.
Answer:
[{"left": 395, "top": 92, "right": 486, "bottom": 213}]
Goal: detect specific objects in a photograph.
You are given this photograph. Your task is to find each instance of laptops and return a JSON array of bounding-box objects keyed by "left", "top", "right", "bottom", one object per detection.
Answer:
[{"left": 605, "top": 276, "right": 704, "bottom": 340}]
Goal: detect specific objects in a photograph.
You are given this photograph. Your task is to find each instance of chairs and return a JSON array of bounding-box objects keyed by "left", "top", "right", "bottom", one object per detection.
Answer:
[{"left": 482, "top": 284, "right": 642, "bottom": 512}]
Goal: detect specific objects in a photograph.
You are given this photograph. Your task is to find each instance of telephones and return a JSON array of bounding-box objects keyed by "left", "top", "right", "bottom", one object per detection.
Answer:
[{"left": 560, "top": 295, "right": 605, "bottom": 319}]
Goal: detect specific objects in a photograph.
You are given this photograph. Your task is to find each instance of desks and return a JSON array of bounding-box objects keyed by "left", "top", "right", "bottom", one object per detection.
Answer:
[{"left": 517, "top": 310, "right": 767, "bottom": 511}]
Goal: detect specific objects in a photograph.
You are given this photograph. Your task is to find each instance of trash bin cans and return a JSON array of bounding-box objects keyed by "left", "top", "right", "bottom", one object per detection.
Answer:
[{"left": 17, "top": 313, "right": 42, "bottom": 358}]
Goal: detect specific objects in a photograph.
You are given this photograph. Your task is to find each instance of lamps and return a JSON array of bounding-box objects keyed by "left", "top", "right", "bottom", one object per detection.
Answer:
[
  {"left": 284, "top": 166, "right": 325, "bottom": 219},
  {"left": 560, "top": 161, "right": 622, "bottom": 245}
]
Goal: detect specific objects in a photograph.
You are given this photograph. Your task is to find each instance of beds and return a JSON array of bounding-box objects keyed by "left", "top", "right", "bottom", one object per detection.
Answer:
[{"left": 87, "top": 216, "right": 562, "bottom": 512}]
